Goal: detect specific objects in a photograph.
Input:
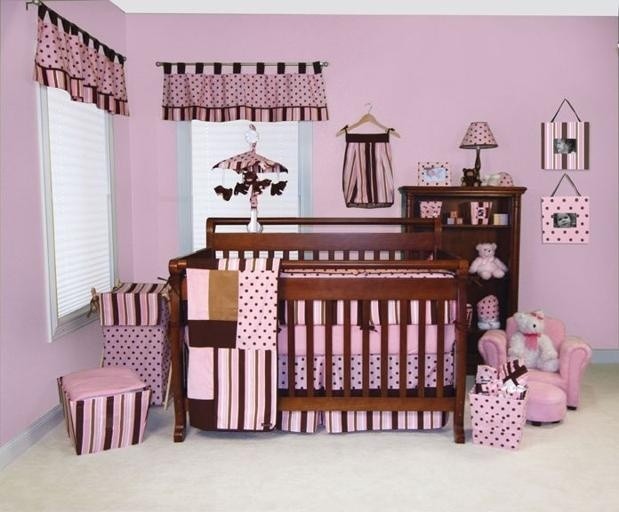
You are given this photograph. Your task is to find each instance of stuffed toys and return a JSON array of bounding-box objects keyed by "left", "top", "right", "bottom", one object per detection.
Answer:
[
  {"left": 460, "top": 167, "right": 483, "bottom": 187},
  {"left": 468, "top": 242, "right": 510, "bottom": 279},
  {"left": 211, "top": 177, "right": 289, "bottom": 203},
  {"left": 508, "top": 309, "right": 559, "bottom": 373}
]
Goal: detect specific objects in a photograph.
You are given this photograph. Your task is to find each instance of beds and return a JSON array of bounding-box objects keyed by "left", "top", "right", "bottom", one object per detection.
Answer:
[{"left": 169, "top": 216, "right": 470, "bottom": 445}]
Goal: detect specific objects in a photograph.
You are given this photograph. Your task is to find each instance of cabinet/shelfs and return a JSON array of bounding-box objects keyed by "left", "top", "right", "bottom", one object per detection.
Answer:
[{"left": 398, "top": 185, "right": 527, "bottom": 375}]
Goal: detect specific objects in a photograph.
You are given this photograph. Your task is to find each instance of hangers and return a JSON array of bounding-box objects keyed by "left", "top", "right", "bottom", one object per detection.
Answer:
[{"left": 335, "top": 102, "right": 400, "bottom": 138}]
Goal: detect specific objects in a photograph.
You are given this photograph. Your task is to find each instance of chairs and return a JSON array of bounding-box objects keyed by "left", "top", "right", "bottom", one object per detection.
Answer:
[{"left": 477, "top": 314, "right": 593, "bottom": 411}]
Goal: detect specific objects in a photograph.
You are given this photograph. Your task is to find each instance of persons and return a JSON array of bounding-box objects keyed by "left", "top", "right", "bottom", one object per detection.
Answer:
[
  {"left": 555, "top": 138, "right": 577, "bottom": 154},
  {"left": 552, "top": 213, "right": 577, "bottom": 230}
]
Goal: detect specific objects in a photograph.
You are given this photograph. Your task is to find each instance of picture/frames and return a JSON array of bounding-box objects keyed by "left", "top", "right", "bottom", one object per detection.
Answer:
[{"left": 417, "top": 161, "right": 451, "bottom": 187}]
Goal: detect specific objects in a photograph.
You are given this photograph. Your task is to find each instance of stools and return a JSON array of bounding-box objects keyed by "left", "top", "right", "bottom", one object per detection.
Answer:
[
  {"left": 57, "top": 366, "right": 152, "bottom": 455},
  {"left": 523, "top": 382, "right": 566, "bottom": 427}
]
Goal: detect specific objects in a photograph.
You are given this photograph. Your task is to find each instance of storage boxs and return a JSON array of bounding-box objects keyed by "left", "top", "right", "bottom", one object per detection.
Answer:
[
  {"left": 465, "top": 200, "right": 493, "bottom": 225},
  {"left": 416, "top": 200, "right": 443, "bottom": 218},
  {"left": 468, "top": 381, "right": 529, "bottom": 451}
]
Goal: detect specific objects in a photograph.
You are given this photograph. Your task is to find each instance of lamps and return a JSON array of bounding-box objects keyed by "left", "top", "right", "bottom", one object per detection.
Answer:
[{"left": 459, "top": 121, "right": 498, "bottom": 186}]
